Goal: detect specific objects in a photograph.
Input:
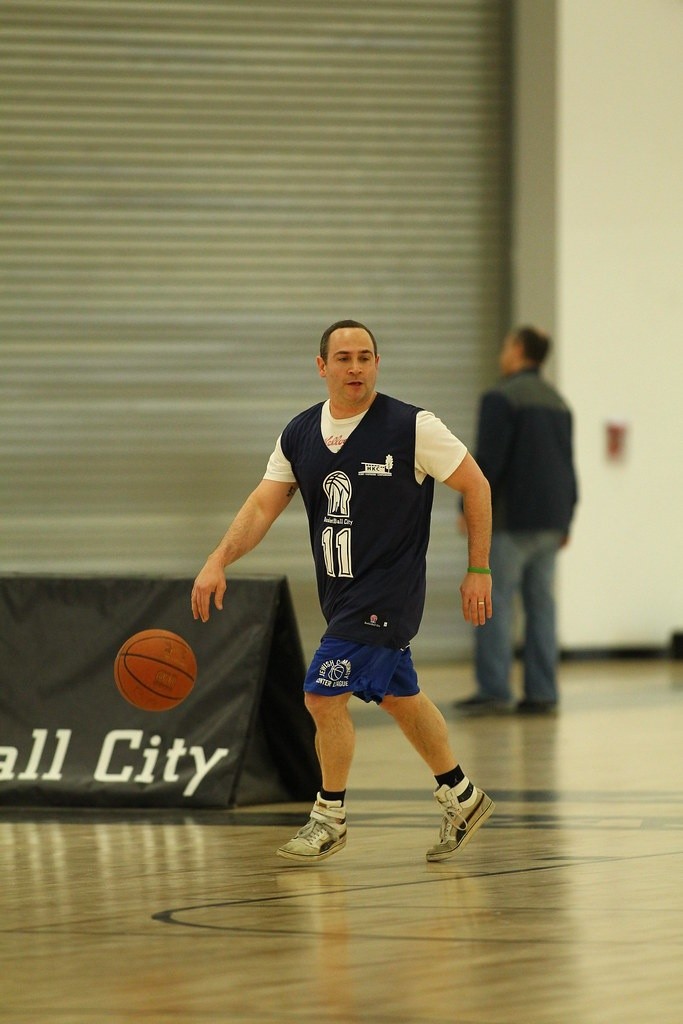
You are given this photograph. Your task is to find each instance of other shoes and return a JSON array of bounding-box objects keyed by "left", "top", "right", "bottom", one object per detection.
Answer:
[
  {"left": 454, "top": 693, "right": 516, "bottom": 717},
  {"left": 516, "top": 701, "right": 559, "bottom": 719}
]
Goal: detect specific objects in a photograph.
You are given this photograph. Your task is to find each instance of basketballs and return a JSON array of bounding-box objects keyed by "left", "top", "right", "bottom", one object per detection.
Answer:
[{"left": 113, "top": 627, "right": 197, "bottom": 711}]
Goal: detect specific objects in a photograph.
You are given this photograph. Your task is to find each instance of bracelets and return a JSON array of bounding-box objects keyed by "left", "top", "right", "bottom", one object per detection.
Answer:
[{"left": 467, "top": 567, "right": 491, "bottom": 574}]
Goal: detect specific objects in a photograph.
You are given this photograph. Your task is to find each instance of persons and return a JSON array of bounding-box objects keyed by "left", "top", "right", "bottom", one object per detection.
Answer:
[
  {"left": 450, "top": 324, "right": 578, "bottom": 716},
  {"left": 189, "top": 320, "right": 496, "bottom": 862}
]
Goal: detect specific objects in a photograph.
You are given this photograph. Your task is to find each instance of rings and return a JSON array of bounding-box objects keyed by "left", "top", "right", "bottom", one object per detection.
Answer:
[{"left": 478, "top": 601, "right": 485, "bottom": 604}]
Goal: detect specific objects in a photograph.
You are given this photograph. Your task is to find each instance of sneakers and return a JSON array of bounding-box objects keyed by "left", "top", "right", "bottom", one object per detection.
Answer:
[
  {"left": 275, "top": 792, "right": 347, "bottom": 862},
  {"left": 426, "top": 775, "right": 496, "bottom": 862}
]
